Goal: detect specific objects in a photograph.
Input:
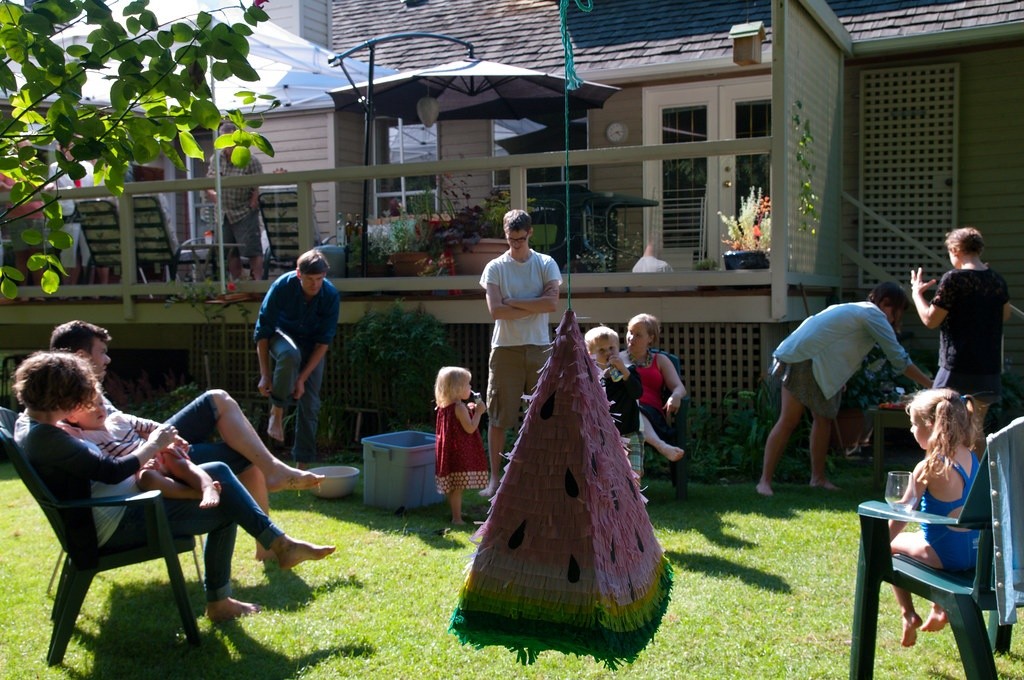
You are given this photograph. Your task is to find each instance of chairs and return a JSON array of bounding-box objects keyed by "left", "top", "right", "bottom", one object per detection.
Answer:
[
  {"left": 848, "top": 416, "right": 1024, "bottom": 680},
  {"left": 0, "top": 407, "right": 206, "bottom": 668},
  {"left": 254, "top": 192, "right": 320, "bottom": 281},
  {"left": 638, "top": 351, "right": 690, "bottom": 500},
  {"left": 76, "top": 196, "right": 174, "bottom": 300}
]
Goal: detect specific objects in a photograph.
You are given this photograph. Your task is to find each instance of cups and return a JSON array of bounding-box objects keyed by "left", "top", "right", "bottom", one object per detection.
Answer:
[
  {"left": 204, "top": 230, "right": 212, "bottom": 245},
  {"left": 885, "top": 470, "right": 918, "bottom": 513}
]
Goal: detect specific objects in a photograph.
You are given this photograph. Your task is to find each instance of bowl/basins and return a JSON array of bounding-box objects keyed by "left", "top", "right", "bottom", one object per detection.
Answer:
[{"left": 307, "top": 466, "right": 360, "bottom": 498}]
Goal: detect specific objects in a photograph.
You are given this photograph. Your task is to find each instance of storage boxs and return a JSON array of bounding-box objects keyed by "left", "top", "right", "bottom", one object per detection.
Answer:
[{"left": 361, "top": 431, "right": 446, "bottom": 510}]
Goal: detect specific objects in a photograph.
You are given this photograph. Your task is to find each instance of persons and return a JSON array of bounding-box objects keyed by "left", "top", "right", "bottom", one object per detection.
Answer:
[
  {"left": 62, "top": 384, "right": 220, "bottom": 507},
  {"left": 205, "top": 122, "right": 264, "bottom": 282},
  {"left": 0, "top": 137, "right": 180, "bottom": 286},
  {"left": 632, "top": 222, "right": 691, "bottom": 291},
  {"left": 910, "top": 227, "right": 1011, "bottom": 433},
  {"left": 432, "top": 366, "right": 486, "bottom": 525},
  {"left": 619, "top": 313, "right": 687, "bottom": 461},
  {"left": 478, "top": 210, "right": 563, "bottom": 497},
  {"left": 584, "top": 322, "right": 645, "bottom": 478},
  {"left": 10, "top": 347, "right": 335, "bottom": 619},
  {"left": 888, "top": 387, "right": 985, "bottom": 648},
  {"left": 51, "top": 320, "right": 325, "bottom": 561},
  {"left": 254, "top": 248, "right": 340, "bottom": 471},
  {"left": 755, "top": 282, "right": 935, "bottom": 497}
]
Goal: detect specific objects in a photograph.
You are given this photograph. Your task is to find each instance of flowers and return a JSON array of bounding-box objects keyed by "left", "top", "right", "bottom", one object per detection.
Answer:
[
  {"left": 714, "top": 186, "right": 771, "bottom": 253},
  {"left": 829, "top": 342, "right": 933, "bottom": 413},
  {"left": 441, "top": 175, "right": 531, "bottom": 248}
]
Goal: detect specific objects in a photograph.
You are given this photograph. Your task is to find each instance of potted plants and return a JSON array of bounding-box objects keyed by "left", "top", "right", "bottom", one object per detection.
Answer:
[{"left": 372, "top": 185, "right": 456, "bottom": 275}]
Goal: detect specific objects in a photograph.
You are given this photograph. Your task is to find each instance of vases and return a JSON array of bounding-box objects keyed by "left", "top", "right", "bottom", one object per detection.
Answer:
[
  {"left": 14, "top": 251, "right": 119, "bottom": 285},
  {"left": 830, "top": 406, "right": 877, "bottom": 453},
  {"left": 448, "top": 237, "right": 510, "bottom": 275},
  {"left": 721, "top": 251, "right": 766, "bottom": 271}
]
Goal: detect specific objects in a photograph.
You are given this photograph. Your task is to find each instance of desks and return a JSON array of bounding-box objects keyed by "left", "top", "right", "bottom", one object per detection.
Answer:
[
  {"left": 179, "top": 243, "right": 245, "bottom": 281},
  {"left": 874, "top": 403, "right": 914, "bottom": 484}
]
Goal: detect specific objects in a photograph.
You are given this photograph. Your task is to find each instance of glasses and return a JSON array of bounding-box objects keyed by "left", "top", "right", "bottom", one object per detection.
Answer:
[{"left": 507, "top": 232, "right": 528, "bottom": 243}]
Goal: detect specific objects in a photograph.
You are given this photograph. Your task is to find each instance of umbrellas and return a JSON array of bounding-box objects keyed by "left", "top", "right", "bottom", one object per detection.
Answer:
[
  {"left": 0, "top": 0, "right": 399, "bottom": 295},
  {"left": 324, "top": 58, "right": 622, "bottom": 214}
]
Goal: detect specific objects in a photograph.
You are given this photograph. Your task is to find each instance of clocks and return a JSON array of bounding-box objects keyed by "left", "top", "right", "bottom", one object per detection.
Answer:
[{"left": 606, "top": 122, "right": 628, "bottom": 145}]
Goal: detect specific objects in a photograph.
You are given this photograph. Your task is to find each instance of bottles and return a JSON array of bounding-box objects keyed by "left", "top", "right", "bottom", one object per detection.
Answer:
[{"left": 474, "top": 392, "right": 482, "bottom": 405}]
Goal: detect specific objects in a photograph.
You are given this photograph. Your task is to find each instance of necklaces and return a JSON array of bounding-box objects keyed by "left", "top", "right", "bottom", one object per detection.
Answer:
[{"left": 626, "top": 348, "right": 652, "bottom": 367}]
